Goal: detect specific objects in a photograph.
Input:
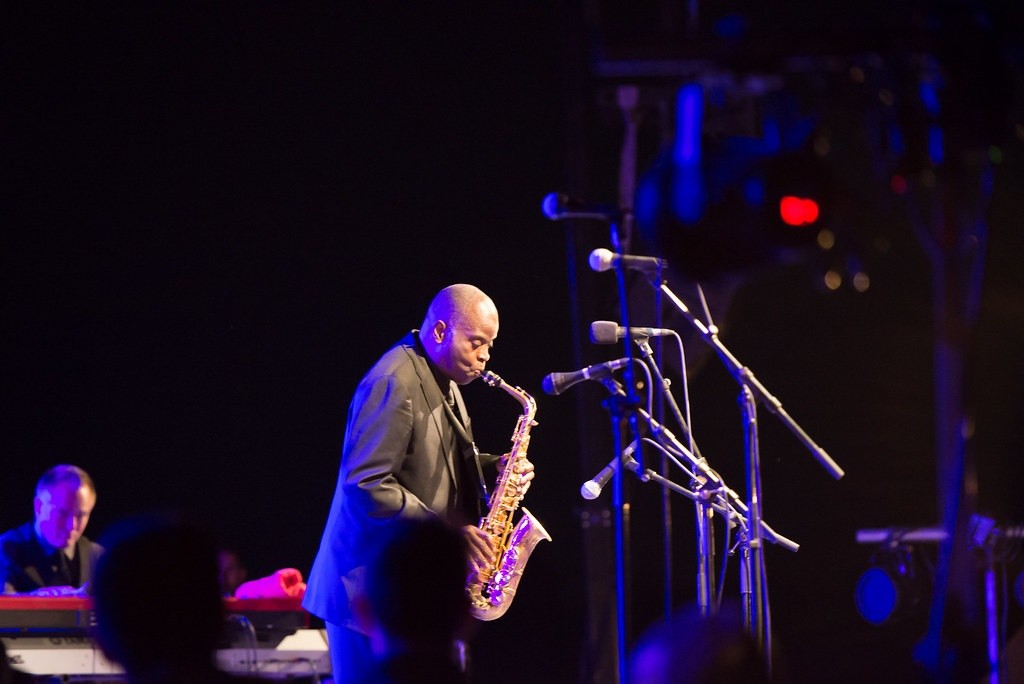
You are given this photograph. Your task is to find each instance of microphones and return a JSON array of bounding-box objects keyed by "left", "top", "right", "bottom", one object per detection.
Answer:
[
  {"left": 542, "top": 358, "right": 631, "bottom": 396},
  {"left": 581, "top": 441, "right": 637, "bottom": 500},
  {"left": 543, "top": 192, "right": 629, "bottom": 222},
  {"left": 588, "top": 248, "right": 669, "bottom": 272},
  {"left": 590, "top": 320, "right": 674, "bottom": 345}
]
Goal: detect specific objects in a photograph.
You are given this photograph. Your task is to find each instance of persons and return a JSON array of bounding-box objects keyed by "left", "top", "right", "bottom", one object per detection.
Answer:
[
  {"left": 301, "top": 283, "right": 535, "bottom": 684},
  {"left": 0, "top": 466, "right": 298, "bottom": 684},
  {"left": 627, "top": 571, "right": 1023, "bottom": 684}
]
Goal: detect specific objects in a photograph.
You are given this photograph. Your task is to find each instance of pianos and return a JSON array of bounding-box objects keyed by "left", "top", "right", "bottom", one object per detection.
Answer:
[{"left": 0, "top": 581, "right": 320, "bottom": 649}]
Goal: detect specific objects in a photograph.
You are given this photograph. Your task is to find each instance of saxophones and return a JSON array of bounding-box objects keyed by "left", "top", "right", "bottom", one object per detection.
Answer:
[{"left": 454, "top": 366, "right": 560, "bottom": 627}]
